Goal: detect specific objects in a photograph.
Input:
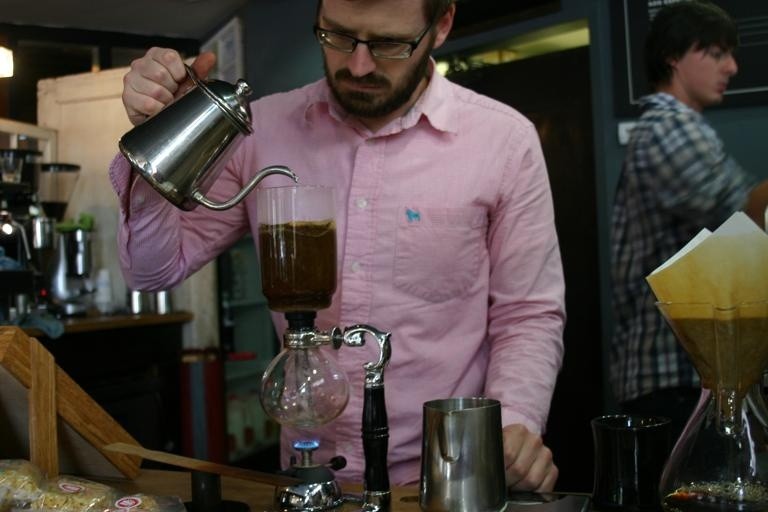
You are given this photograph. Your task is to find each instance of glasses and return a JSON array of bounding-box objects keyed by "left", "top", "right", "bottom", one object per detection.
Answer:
[{"left": 310, "top": 17, "right": 431, "bottom": 60}]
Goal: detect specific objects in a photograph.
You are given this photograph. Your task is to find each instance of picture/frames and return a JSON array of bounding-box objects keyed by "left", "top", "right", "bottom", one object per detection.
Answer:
[{"left": 610, "top": 0, "right": 768, "bottom": 118}]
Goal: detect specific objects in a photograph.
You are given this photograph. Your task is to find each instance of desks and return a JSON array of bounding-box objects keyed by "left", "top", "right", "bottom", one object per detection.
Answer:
[{"left": 22, "top": 310, "right": 194, "bottom": 472}]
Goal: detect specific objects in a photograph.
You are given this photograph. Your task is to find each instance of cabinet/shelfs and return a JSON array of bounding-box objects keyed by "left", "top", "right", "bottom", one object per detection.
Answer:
[{"left": 216, "top": 232, "right": 282, "bottom": 475}]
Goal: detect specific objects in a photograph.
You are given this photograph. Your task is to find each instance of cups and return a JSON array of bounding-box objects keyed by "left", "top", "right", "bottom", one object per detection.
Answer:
[
  {"left": 418, "top": 396, "right": 507, "bottom": 511},
  {"left": 590, "top": 414, "right": 673, "bottom": 512}
]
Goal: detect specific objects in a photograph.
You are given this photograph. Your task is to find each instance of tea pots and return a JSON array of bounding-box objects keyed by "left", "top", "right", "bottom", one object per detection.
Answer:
[{"left": 117, "top": 64, "right": 300, "bottom": 212}]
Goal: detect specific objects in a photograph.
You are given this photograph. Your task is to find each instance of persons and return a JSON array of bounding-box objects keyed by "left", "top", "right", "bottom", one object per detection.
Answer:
[
  {"left": 109, "top": 0, "right": 566, "bottom": 495},
  {"left": 604, "top": 2, "right": 768, "bottom": 489}
]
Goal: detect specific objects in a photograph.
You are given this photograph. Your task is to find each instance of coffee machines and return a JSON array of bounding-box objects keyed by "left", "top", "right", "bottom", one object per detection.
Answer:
[{"left": 0, "top": 147, "right": 99, "bottom": 326}]
